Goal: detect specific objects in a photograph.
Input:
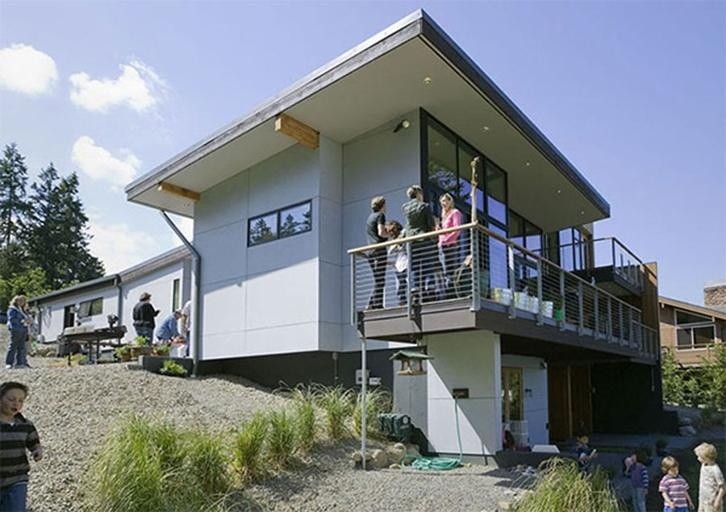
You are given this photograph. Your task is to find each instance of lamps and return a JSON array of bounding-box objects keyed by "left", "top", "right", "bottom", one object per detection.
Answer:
[{"left": 394, "top": 119, "right": 410, "bottom": 133}]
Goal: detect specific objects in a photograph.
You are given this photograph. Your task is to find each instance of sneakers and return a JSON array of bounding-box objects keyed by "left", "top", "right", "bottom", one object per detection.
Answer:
[
  {"left": 5, "top": 363, "right": 13, "bottom": 369},
  {"left": 15, "top": 363, "right": 28, "bottom": 370}
]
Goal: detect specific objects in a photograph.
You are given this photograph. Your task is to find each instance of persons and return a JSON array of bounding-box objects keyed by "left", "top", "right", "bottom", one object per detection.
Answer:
[
  {"left": 179, "top": 297, "right": 192, "bottom": 357},
  {"left": 657, "top": 454, "right": 697, "bottom": 511},
  {"left": 3, "top": 295, "right": 28, "bottom": 368},
  {"left": 438, "top": 192, "right": 463, "bottom": 299},
  {"left": 576, "top": 431, "right": 601, "bottom": 478},
  {"left": 623, "top": 450, "right": 649, "bottom": 511},
  {"left": 399, "top": 184, "right": 446, "bottom": 302},
  {"left": 10, "top": 294, "right": 33, "bottom": 369},
  {"left": 623, "top": 454, "right": 634, "bottom": 478},
  {"left": 0, "top": 380, "right": 43, "bottom": 512},
  {"left": 385, "top": 220, "right": 409, "bottom": 304},
  {"left": 693, "top": 441, "right": 726, "bottom": 512},
  {"left": 155, "top": 307, "right": 183, "bottom": 341},
  {"left": 132, "top": 292, "right": 160, "bottom": 345},
  {"left": 360, "top": 194, "right": 397, "bottom": 311}
]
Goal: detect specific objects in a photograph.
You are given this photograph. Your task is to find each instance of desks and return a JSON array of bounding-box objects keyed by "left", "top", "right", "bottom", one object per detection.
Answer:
[{"left": 56, "top": 325, "right": 128, "bottom": 366}]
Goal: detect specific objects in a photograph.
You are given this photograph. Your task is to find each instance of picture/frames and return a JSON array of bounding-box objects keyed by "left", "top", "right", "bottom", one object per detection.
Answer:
[
  {"left": 278, "top": 199, "right": 312, "bottom": 239},
  {"left": 247, "top": 210, "right": 279, "bottom": 247}
]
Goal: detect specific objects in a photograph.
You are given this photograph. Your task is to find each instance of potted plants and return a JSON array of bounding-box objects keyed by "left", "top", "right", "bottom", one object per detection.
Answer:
[{"left": 114, "top": 335, "right": 170, "bottom": 363}]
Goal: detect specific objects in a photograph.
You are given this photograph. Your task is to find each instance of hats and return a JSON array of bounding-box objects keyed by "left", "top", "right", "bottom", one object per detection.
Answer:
[{"left": 176, "top": 308, "right": 182, "bottom": 317}]
